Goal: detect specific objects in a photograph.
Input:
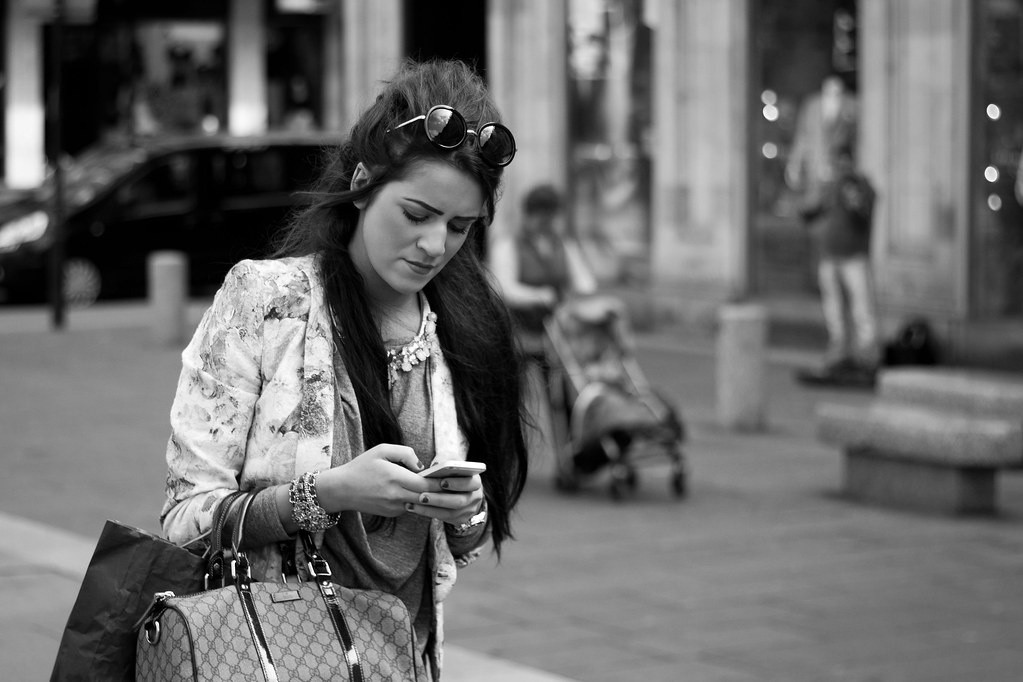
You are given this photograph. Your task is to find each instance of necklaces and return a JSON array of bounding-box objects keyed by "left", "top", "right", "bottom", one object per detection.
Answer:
[{"left": 329, "top": 297, "right": 438, "bottom": 385}]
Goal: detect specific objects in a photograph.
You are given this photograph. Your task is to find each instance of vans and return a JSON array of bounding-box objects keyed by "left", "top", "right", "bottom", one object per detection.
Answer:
[{"left": 0, "top": 134, "right": 346, "bottom": 308}]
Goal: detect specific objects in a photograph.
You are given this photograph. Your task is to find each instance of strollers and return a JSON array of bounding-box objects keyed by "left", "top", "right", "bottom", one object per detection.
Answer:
[{"left": 543, "top": 293, "right": 689, "bottom": 501}]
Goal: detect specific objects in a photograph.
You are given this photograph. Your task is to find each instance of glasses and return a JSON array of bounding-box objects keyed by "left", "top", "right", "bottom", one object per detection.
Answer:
[{"left": 387, "top": 106, "right": 515, "bottom": 166}]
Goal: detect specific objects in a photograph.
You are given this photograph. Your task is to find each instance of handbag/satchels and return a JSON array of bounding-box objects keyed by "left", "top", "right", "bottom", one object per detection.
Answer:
[
  {"left": 50, "top": 489, "right": 225, "bottom": 682},
  {"left": 135, "top": 487, "right": 429, "bottom": 682}
]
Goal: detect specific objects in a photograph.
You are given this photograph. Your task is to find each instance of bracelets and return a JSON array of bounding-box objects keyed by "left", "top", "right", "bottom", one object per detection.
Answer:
[{"left": 287, "top": 470, "right": 341, "bottom": 536}]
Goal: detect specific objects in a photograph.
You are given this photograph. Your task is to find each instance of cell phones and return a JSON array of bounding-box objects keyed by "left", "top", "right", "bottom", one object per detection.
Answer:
[{"left": 419, "top": 461, "right": 486, "bottom": 480}]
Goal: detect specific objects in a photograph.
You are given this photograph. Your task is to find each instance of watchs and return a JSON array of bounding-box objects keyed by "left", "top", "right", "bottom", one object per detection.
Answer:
[{"left": 455, "top": 501, "right": 488, "bottom": 533}]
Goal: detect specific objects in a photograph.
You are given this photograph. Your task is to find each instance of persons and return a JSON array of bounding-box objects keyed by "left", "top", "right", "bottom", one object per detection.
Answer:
[
  {"left": 493, "top": 185, "right": 635, "bottom": 397},
  {"left": 796, "top": 146, "right": 881, "bottom": 370},
  {"left": 778, "top": 73, "right": 857, "bottom": 293},
  {"left": 158, "top": 60, "right": 543, "bottom": 682}
]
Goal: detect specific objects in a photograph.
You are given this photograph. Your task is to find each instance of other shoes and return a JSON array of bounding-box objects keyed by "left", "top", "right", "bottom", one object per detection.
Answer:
[
  {"left": 797, "top": 361, "right": 881, "bottom": 391},
  {"left": 556, "top": 475, "right": 581, "bottom": 492}
]
